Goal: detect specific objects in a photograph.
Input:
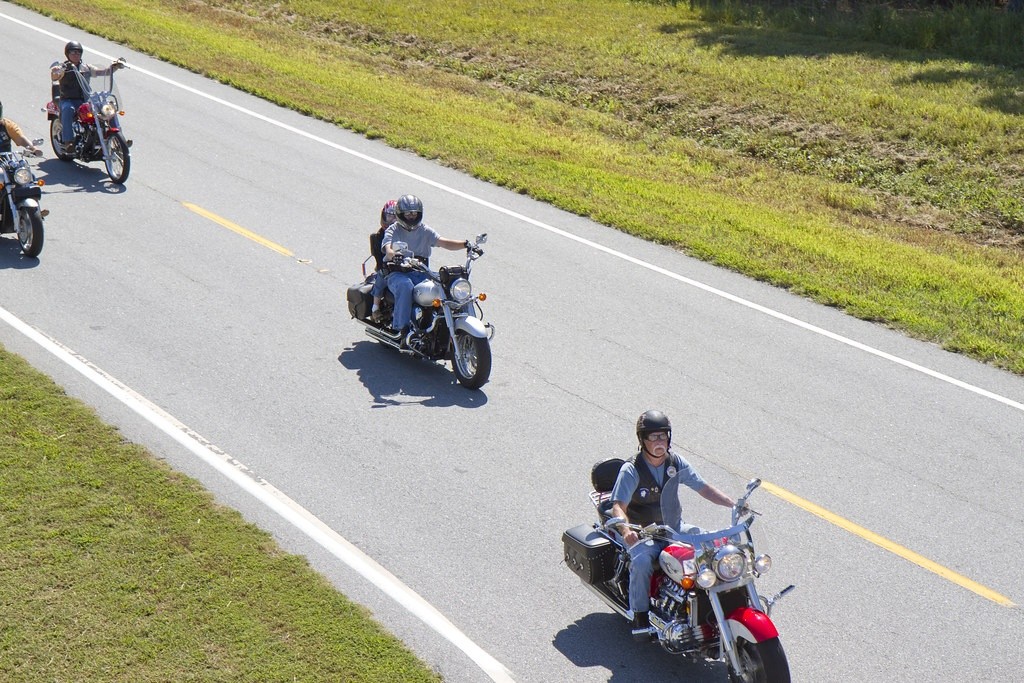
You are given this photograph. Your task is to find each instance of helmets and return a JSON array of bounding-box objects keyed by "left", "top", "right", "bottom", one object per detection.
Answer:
[
  {"left": 382, "top": 200, "right": 400, "bottom": 228},
  {"left": 637, "top": 409, "right": 671, "bottom": 434},
  {"left": 65, "top": 42, "right": 83, "bottom": 58},
  {"left": 396, "top": 195, "right": 423, "bottom": 231}
]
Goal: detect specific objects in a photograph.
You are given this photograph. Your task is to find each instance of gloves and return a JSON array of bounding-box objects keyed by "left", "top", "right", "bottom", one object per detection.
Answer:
[{"left": 465, "top": 241, "right": 476, "bottom": 252}]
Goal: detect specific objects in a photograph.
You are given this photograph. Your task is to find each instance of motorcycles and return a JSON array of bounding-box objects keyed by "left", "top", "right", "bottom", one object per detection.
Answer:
[
  {"left": 562, "top": 458, "right": 795, "bottom": 683},
  {"left": 40, "top": 57, "right": 130, "bottom": 183},
  {"left": 0, "top": 138, "right": 50, "bottom": 258},
  {"left": 347, "top": 233, "right": 495, "bottom": 389}
]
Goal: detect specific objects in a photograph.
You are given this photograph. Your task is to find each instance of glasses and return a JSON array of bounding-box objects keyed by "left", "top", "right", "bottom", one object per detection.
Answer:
[
  {"left": 643, "top": 433, "right": 668, "bottom": 441},
  {"left": 404, "top": 211, "right": 418, "bottom": 216},
  {"left": 68, "top": 50, "right": 82, "bottom": 55}
]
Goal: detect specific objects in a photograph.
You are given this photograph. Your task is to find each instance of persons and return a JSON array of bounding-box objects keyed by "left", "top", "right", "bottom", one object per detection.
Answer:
[
  {"left": 608, "top": 409, "right": 749, "bottom": 633},
  {"left": 369, "top": 194, "right": 473, "bottom": 348},
  {"left": 0, "top": 101, "right": 43, "bottom": 165},
  {"left": 48, "top": 41, "right": 126, "bottom": 152}
]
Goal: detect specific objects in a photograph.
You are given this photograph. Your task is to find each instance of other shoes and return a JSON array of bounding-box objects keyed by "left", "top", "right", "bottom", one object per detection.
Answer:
[
  {"left": 401, "top": 328, "right": 412, "bottom": 348},
  {"left": 633, "top": 611, "right": 649, "bottom": 629},
  {"left": 66, "top": 142, "right": 74, "bottom": 152},
  {"left": 372, "top": 309, "right": 384, "bottom": 322}
]
